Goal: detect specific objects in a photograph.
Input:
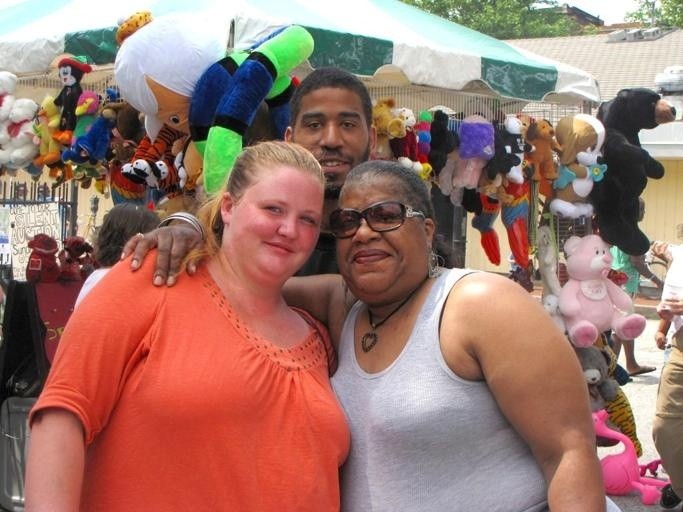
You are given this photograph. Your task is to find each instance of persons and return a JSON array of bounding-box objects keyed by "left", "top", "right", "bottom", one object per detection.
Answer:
[
  {"left": 643, "top": 230, "right": 683, "bottom": 512},
  {"left": 646, "top": 243, "right": 677, "bottom": 267},
  {"left": 14, "top": 139, "right": 351, "bottom": 509},
  {"left": 267, "top": 69, "right": 389, "bottom": 277},
  {"left": 608, "top": 198, "right": 658, "bottom": 377},
  {"left": 116, "top": 159, "right": 605, "bottom": 510}
]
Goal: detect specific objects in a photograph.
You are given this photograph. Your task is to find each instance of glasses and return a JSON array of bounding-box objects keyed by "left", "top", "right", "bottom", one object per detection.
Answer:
[{"left": 327, "top": 200, "right": 422, "bottom": 239}]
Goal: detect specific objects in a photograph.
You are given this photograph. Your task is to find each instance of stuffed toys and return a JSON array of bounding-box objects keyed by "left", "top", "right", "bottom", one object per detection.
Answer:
[
  {"left": 596, "top": 332, "right": 645, "bottom": 460},
  {"left": 371, "top": 90, "right": 676, "bottom": 350},
  {"left": 57, "top": 235, "right": 92, "bottom": 283},
  {"left": 26, "top": 234, "right": 67, "bottom": 286},
  {"left": 592, "top": 407, "right": 670, "bottom": 505},
  {"left": 572, "top": 345, "right": 616, "bottom": 412}
]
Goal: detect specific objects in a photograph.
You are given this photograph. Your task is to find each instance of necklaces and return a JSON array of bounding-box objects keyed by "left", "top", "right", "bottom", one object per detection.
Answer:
[{"left": 360, "top": 275, "right": 428, "bottom": 353}]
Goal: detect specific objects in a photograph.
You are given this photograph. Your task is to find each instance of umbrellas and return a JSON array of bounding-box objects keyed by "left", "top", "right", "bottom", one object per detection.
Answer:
[{"left": 0, "top": 1, "right": 602, "bottom": 120}]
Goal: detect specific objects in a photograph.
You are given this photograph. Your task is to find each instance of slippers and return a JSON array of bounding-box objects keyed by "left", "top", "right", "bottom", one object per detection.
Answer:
[{"left": 626, "top": 365, "right": 657, "bottom": 376}]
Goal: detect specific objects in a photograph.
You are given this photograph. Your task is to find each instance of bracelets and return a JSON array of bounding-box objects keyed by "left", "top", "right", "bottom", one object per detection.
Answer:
[
  {"left": 646, "top": 272, "right": 656, "bottom": 281},
  {"left": 155, "top": 210, "right": 207, "bottom": 245}
]
